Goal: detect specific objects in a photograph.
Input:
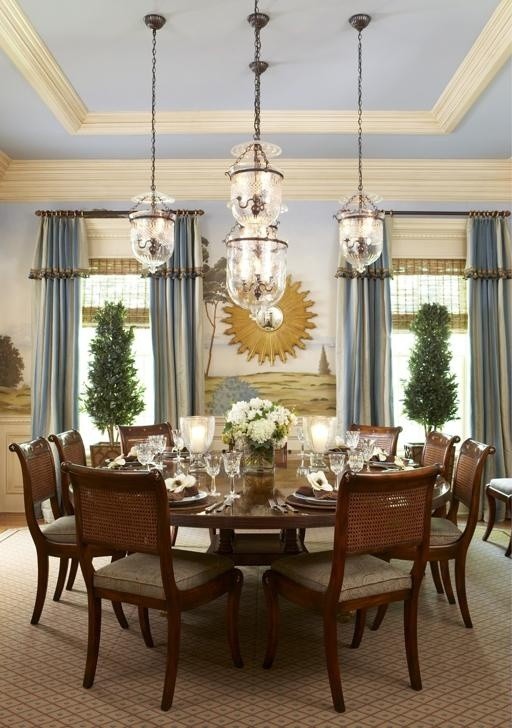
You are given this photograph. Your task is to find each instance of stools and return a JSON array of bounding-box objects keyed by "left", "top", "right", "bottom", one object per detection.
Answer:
[{"left": 481, "top": 476, "right": 512, "bottom": 558}]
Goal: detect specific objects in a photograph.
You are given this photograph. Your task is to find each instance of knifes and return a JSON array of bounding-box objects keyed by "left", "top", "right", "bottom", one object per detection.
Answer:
[
  {"left": 218, "top": 496, "right": 236, "bottom": 513},
  {"left": 266, "top": 492, "right": 288, "bottom": 516},
  {"left": 206, "top": 499, "right": 222, "bottom": 513},
  {"left": 276, "top": 492, "right": 299, "bottom": 514}
]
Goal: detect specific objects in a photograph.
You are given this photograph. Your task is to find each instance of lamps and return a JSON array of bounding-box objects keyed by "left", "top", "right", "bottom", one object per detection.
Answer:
[
  {"left": 335, "top": 14, "right": 386, "bottom": 273},
  {"left": 222, "top": 1, "right": 285, "bottom": 230},
  {"left": 128, "top": 13, "right": 176, "bottom": 274},
  {"left": 223, "top": 60, "right": 288, "bottom": 319}
]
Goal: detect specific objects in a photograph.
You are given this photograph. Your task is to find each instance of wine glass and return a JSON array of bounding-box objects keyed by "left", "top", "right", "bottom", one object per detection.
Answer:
[
  {"left": 172, "top": 431, "right": 184, "bottom": 465},
  {"left": 329, "top": 454, "right": 343, "bottom": 489},
  {"left": 222, "top": 452, "right": 243, "bottom": 499},
  {"left": 148, "top": 435, "right": 167, "bottom": 468},
  {"left": 362, "top": 438, "right": 378, "bottom": 472},
  {"left": 345, "top": 430, "right": 360, "bottom": 449},
  {"left": 297, "top": 426, "right": 309, "bottom": 473},
  {"left": 137, "top": 448, "right": 155, "bottom": 466},
  {"left": 203, "top": 456, "right": 222, "bottom": 496},
  {"left": 347, "top": 450, "right": 364, "bottom": 473}
]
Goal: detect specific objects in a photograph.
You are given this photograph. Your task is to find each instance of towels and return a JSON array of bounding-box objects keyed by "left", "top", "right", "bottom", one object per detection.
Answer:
[
  {"left": 164, "top": 483, "right": 199, "bottom": 501},
  {"left": 328, "top": 447, "right": 351, "bottom": 452},
  {"left": 297, "top": 486, "right": 338, "bottom": 500},
  {"left": 371, "top": 455, "right": 395, "bottom": 461},
  {"left": 109, "top": 455, "right": 140, "bottom": 463}
]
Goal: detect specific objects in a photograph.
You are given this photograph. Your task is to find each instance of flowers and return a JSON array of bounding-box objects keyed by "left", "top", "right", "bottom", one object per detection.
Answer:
[{"left": 222, "top": 397, "right": 296, "bottom": 465}]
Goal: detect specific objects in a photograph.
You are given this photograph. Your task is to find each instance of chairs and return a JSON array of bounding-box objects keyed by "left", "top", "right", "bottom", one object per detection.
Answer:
[
  {"left": 49, "top": 428, "right": 126, "bottom": 591},
  {"left": 9, "top": 437, "right": 129, "bottom": 630},
  {"left": 261, "top": 462, "right": 446, "bottom": 713},
  {"left": 340, "top": 423, "right": 402, "bottom": 461},
  {"left": 369, "top": 437, "right": 497, "bottom": 631},
  {"left": 61, "top": 461, "right": 245, "bottom": 712},
  {"left": 378, "top": 432, "right": 460, "bottom": 562},
  {"left": 116, "top": 423, "right": 175, "bottom": 461}
]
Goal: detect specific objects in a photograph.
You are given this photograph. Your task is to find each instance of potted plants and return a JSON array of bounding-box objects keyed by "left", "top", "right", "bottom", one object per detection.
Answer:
[
  {"left": 399, "top": 302, "right": 460, "bottom": 465},
  {"left": 76, "top": 297, "right": 148, "bottom": 466}
]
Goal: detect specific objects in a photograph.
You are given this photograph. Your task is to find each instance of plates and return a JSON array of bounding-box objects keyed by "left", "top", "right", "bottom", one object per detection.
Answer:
[
  {"left": 293, "top": 484, "right": 337, "bottom": 505},
  {"left": 168, "top": 488, "right": 206, "bottom": 507},
  {"left": 285, "top": 494, "right": 337, "bottom": 515}
]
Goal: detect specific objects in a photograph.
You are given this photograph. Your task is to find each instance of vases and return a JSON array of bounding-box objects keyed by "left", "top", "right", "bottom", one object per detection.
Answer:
[{"left": 238, "top": 440, "right": 276, "bottom": 470}]
